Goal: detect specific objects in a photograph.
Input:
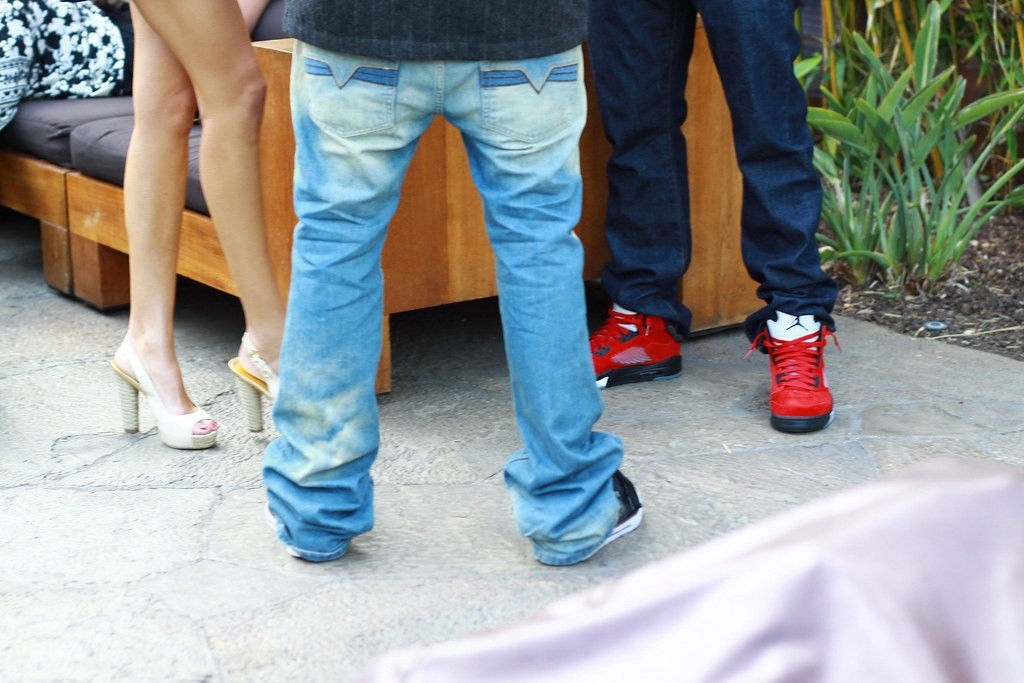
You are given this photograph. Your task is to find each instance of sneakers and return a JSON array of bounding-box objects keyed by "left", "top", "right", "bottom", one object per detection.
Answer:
[
  {"left": 742, "top": 311, "right": 842, "bottom": 432},
  {"left": 581, "top": 468, "right": 642, "bottom": 563},
  {"left": 589, "top": 302, "right": 682, "bottom": 390}
]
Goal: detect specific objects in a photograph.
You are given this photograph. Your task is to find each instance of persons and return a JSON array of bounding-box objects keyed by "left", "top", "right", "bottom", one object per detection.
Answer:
[
  {"left": 262, "top": 0, "right": 643, "bottom": 565},
  {"left": 113, "top": 0, "right": 282, "bottom": 450},
  {"left": 0, "top": 0, "right": 271, "bottom": 131},
  {"left": 582, "top": 0, "right": 841, "bottom": 432}
]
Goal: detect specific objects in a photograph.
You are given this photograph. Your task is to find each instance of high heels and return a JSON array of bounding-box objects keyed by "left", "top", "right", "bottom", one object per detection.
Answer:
[
  {"left": 228, "top": 332, "right": 280, "bottom": 433},
  {"left": 110, "top": 339, "right": 217, "bottom": 449}
]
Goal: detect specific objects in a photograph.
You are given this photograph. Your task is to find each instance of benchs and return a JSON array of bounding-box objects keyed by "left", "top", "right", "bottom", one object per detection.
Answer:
[{"left": 0, "top": 0, "right": 624, "bottom": 400}]
